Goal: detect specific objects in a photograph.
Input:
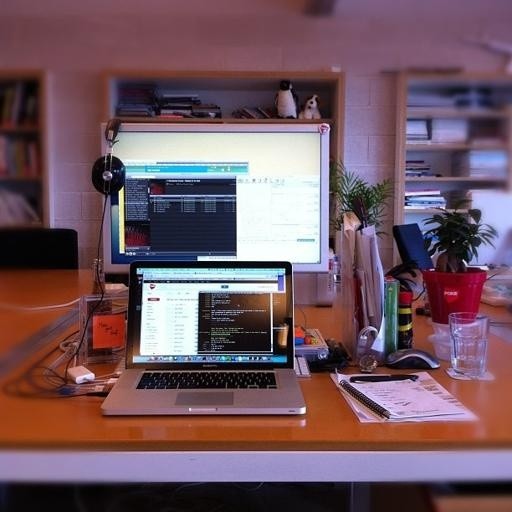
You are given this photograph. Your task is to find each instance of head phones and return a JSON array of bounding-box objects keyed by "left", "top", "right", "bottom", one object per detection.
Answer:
[{"left": 91, "top": 120, "right": 126, "bottom": 193}]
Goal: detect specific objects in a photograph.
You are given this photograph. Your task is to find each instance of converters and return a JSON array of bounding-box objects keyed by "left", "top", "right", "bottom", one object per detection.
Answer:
[{"left": 67, "top": 365, "right": 95, "bottom": 385}]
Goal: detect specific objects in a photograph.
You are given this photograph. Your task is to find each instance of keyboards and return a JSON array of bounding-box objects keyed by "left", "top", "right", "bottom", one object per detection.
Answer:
[{"left": 114, "top": 351, "right": 313, "bottom": 378}]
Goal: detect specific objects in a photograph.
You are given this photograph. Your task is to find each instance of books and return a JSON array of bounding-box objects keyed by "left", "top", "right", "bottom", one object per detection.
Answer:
[
  {"left": 116, "top": 82, "right": 222, "bottom": 119},
  {"left": 0, "top": 77, "right": 39, "bottom": 227},
  {"left": 404, "top": 119, "right": 508, "bottom": 210},
  {"left": 330, "top": 366, "right": 479, "bottom": 424}
]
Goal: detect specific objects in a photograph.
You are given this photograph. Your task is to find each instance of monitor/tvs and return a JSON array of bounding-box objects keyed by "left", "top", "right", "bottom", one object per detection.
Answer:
[{"left": 99, "top": 120, "right": 331, "bottom": 275}]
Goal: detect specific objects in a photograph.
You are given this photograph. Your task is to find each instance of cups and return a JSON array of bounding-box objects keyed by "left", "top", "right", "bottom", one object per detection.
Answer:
[{"left": 448, "top": 311, "right": 489, "bottom": 378}]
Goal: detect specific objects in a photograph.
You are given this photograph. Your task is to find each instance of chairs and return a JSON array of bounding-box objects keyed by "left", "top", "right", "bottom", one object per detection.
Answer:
[{"left": 0, "top": 224, "right": 82, "bottom": 271}]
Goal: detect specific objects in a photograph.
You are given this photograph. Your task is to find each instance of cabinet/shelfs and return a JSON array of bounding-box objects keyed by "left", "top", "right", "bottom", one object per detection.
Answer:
[
  {"left": 1, "top": 64, "right": 51, "bottom": 230},
  {"left": 98, "top": 68, "right": 343, "bottom": 264},
  {"left": 388, "top": 70, "right": 511, "bottom": 292}
]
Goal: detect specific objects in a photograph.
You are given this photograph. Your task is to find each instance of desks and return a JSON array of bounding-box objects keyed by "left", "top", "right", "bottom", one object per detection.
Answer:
[{"left": 0, "top": 267, "right": 512, "bottom": 512}]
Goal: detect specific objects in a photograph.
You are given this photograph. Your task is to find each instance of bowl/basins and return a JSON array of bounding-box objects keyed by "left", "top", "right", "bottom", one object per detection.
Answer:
[{"left": 427, "top": 317, "right": 488, "bottom": 363}]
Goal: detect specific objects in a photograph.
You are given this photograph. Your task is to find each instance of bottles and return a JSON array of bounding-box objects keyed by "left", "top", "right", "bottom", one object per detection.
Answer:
[
  {"left": 399, "top": 288, "right": 414, "bottom": 351},
  {"left": 87, "top": 259, "right": 114, "bottom": 356},
  {"left": 384, "top": 275, "right": 399, "bottom": 352}
]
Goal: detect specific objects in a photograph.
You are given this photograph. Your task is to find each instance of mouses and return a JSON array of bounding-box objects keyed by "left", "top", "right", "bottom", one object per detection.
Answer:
[{"left": 385, "top": 348, "right": 439, "bottom": 371}]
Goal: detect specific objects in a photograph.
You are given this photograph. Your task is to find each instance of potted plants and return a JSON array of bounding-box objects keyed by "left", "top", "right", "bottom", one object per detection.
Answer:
[{"left": 419, "top": 195, "right": 498, "bottom": 323}]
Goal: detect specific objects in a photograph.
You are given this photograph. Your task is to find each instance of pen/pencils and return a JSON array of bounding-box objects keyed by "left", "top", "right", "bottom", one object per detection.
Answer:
[{"left": 350, "top": 374, "right": 419, "bottom": 382}]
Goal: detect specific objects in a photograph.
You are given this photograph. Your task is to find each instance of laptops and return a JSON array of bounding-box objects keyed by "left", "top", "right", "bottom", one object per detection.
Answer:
[{"left": 101, "top": 259, "right": 308, "bottom": 418}]
